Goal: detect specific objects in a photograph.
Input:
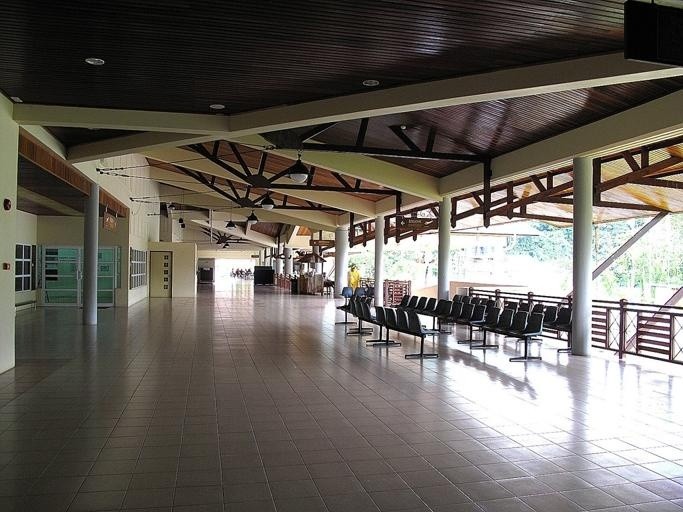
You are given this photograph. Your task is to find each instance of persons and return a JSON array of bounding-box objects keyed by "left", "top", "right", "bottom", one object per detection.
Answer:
[
  {"left": 347, "top": 263, "right": 361, "bottom": 295},
  {"left": 229, "top": 265, "right": 331, "bottom": 293}
]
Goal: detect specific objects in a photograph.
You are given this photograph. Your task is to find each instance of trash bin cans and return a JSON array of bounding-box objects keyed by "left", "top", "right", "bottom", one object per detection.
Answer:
[{"left": 291, "top": 279, "right": 296, "bottom": 294}]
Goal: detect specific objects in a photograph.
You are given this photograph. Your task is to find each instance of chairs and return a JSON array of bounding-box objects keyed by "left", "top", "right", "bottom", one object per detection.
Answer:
[
  {"left": 230, "top": 271, "right": 254, "bottom": 280},
  {"left": 336, "top": 287, "right": 572, "bottom": 359}
]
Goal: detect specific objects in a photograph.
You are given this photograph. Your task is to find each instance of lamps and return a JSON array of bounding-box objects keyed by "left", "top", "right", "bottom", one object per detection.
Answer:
[
  {"left": 248, "top": 210, "right": 258, "bottom": 225},
  {"left": 168, "top": 205, "right": 175, "bottom": 210},
  {"left": 226, "top": 201, "right": 236, "bottom": 230},
  {"left": 179, "top": 190, "right": 185, "bottom": 228},
  {"left": 289, "top": 150, "right": 309, "bottom": 183},
  {"left": 259, "top": 191, "right": 275, "bottom": 210}
]
